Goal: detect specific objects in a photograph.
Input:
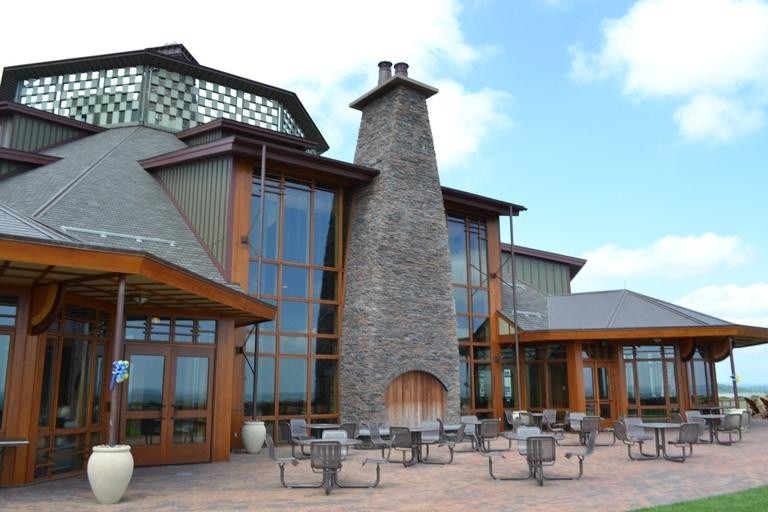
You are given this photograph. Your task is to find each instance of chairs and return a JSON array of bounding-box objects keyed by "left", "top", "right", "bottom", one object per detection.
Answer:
[
  {"left": 265, "top": 418, "right": 466, "bottom": 497},
  {"left": 612, "top": 407, "right": 751, "bottom": 464},
  {"left": 436, "top": 406, "right": 618, "bottom": 487}
]
